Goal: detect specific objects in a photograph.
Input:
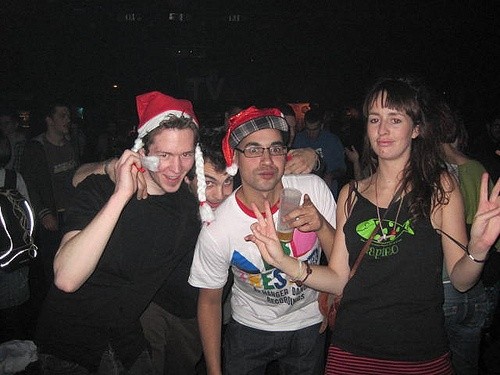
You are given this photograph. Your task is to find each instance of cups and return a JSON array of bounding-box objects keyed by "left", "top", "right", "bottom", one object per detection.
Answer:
[{"left": 276, "top": 188, "right": 302, "bottom": 243}]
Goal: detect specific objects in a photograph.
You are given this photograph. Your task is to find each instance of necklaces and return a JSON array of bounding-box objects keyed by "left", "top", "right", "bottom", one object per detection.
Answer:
[{"left": 376, "top": 171, "right": 405, "bottom": 243}]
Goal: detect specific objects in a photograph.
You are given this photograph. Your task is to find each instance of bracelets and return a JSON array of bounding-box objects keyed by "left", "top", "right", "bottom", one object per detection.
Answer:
[
  {"left": 288, "top": 260, "right": 312, "bottom": 286},
  {"left": 314, "top": 147, "right": 325, "bottom": 174},
  {"left": 466, "top": 244, "right": 491, "bottom": 263},
  {"left": 104, "top": 157, "right": 113, "bottom": 175}
]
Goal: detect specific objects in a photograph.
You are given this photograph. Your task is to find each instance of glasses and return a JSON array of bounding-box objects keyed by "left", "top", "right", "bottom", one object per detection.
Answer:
[{"left": 234, "top": 145, "right": 288, "bottom": 158}]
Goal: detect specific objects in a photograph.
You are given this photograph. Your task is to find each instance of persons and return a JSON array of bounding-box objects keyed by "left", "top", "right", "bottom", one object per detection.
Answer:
[
  {"left": 275, "top": 88, "right": 377, "bottom": 204},
  {"left": 244, "top": 77, "right": 500, "bottom": 375},
  {"left": 71, "top": 141, "right": 319, "bottom": 374},
  {"left": 185, "top": 105, "right": 337, "bottom": 375},
  {"left": 420, "top": 98, "right": 500, "bottom": 375},
  {"left": 35, "top": 91, "right": 234, "bottom": 375},
  {"left": 20, "top": 99, "right": 79, "bottom": 316},
  {"left": 0, "top": 93, "right": 135, "bottom": 172},
  {"left": 0, "top": 132, "right": 36, "bottom": 339}
]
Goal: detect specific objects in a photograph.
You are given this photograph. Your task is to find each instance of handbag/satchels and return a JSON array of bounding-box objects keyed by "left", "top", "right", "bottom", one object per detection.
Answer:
[{"left": 320, "top": 290, "right": 343, "bottom": 334}]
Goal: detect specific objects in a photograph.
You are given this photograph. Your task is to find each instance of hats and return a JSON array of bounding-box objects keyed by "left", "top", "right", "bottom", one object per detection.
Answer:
[
  {"left": 222, "top": 106, "right": 291, "bottom": 177},
  {"left": 135, "top": 91, "right": 200, "bottom": 139}
]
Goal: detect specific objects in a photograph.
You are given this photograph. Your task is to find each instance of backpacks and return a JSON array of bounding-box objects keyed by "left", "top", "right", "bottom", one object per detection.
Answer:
[{"left": 0, "top": 168, "right": 39, "bottom": 274}]
